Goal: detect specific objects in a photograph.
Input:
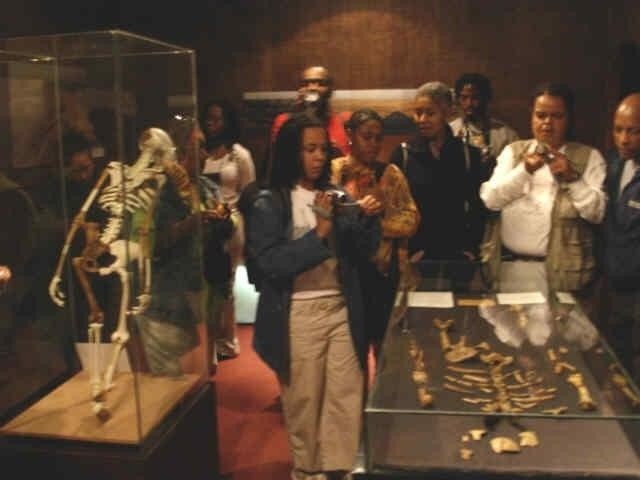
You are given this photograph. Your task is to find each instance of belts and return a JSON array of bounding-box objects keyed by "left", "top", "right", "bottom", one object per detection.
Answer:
[{"left": 499, "top": 248, "right": 545, "bottom": 263}]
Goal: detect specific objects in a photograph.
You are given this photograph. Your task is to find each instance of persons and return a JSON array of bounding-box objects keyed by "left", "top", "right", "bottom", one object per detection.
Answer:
[
  {"left": 51, "top": 78, "right": 131, "bottom": 173},
  {"left": 237, "top": 114, "right": 386, "bottom": 480},
  {"left": 597, "top": 92, "right": 640, "bottom": 371},
  {"left": 1, "top": 175, "right": 40, "bottom": 387},
  {"left": 50, "top": 144, "right": 123, "bottom": 354},
  {"left": 479, "top": 82, "right": 611, "bottom": 291},
  {"left": 155, "top": 65, "right": 519, "bottom": 412}
]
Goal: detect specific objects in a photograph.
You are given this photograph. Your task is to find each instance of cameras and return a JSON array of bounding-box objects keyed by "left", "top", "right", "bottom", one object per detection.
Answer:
[
  {"left": 324, "top": 188, "right": 360, "bottom": 217},
  {"left": 536, "top": 144, "right": 557, "bottom": 160}
]
